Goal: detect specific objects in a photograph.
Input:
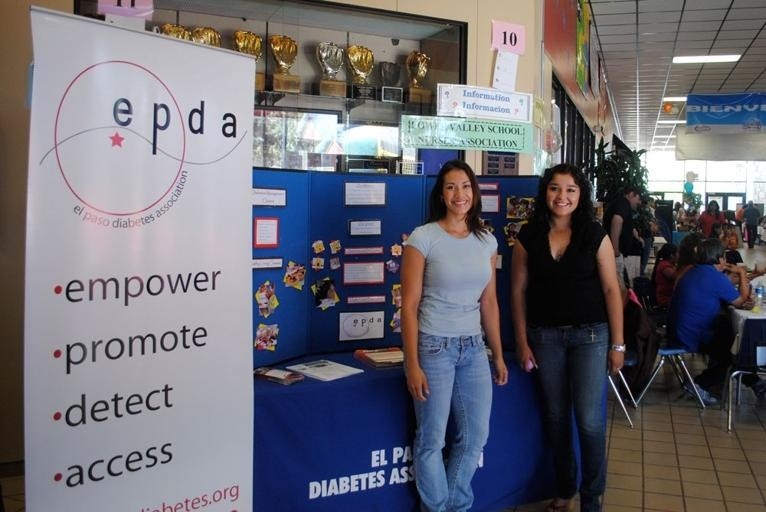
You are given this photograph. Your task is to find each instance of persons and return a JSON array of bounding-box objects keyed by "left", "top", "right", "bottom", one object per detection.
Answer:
[
  {"left": 397, "top": 159, "right": 510, "bottom": 510},
  {"left": 511, "top": 162, "right": 626, "bottom": 510},
  {"left": 608, "top": 187, "right": 766, "bottom": 406}
]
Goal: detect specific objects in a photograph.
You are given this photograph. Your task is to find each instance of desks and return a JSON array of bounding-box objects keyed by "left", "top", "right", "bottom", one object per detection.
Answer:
[{"left": 253, "top": 349, "right": 583, "bottom": 512}]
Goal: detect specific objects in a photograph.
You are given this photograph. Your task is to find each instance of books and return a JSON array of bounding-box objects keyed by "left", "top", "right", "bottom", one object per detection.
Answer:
[
  {"left": 349, "top": 344, "right": 405, "bottom": 371},
  {"left": 284, "top": 357, "right": 365, "bottom": 384}
]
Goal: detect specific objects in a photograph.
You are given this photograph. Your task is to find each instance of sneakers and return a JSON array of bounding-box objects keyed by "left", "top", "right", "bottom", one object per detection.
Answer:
[
  {"left": 751, "top": 379, "right": 766, "bottom": 399},
  {"left": 681, "top": 375, "right": 718, "bottom": 406}
]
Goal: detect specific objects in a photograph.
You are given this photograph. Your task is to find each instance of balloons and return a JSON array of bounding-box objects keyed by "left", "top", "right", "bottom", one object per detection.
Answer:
[
  {"left": 684, "top": 183, "right": 694, "bottom": 193},
  {"left": 686, "top": 171, "right": 697, "bottom": 183},
  {"left": 664, "top": 102, "right": 673, "bottom": 113}
]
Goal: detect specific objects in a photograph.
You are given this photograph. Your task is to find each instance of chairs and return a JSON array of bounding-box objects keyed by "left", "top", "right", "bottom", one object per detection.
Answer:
[{"left": 604, "top": 227, "right": 765, "bottom": 432}]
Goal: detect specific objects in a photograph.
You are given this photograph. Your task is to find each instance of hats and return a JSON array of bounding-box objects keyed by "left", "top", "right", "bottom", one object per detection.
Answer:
[{"left": 635, "top": 187, "right": 648, "bottom": 205}]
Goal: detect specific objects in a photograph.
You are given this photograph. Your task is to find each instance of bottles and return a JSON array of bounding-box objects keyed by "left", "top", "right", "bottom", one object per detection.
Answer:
[{"left": 754, "top": 281, "right": 765, "bottom": 311}]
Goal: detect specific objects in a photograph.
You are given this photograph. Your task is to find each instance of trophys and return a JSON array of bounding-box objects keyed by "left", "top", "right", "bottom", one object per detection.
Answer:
[{"left": 160, "top": 19, "right": 433, "bottom": 105}]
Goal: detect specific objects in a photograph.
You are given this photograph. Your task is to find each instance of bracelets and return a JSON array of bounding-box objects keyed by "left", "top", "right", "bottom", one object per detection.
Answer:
[{"left": 609, "top": 342, "right": 628, "bottom": 355}]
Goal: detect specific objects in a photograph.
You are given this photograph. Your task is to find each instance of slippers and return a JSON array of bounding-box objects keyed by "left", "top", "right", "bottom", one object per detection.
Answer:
[{"left": 544, "top": 495, "right": 575, "bottom": 512}]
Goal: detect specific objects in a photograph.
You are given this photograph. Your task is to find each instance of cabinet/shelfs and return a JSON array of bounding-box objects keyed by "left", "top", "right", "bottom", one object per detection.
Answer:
[{"left": 71, "top": 0, "right": 468, "bottom": 106}]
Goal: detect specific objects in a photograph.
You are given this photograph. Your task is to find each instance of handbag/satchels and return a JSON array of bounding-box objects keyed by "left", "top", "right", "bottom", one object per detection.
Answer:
[
  {"left": 632, "top": 276, "right": 661, "bottom": 316},
  {"left": 654, "top": 320, "right": 676, "bottom": 340}
]
